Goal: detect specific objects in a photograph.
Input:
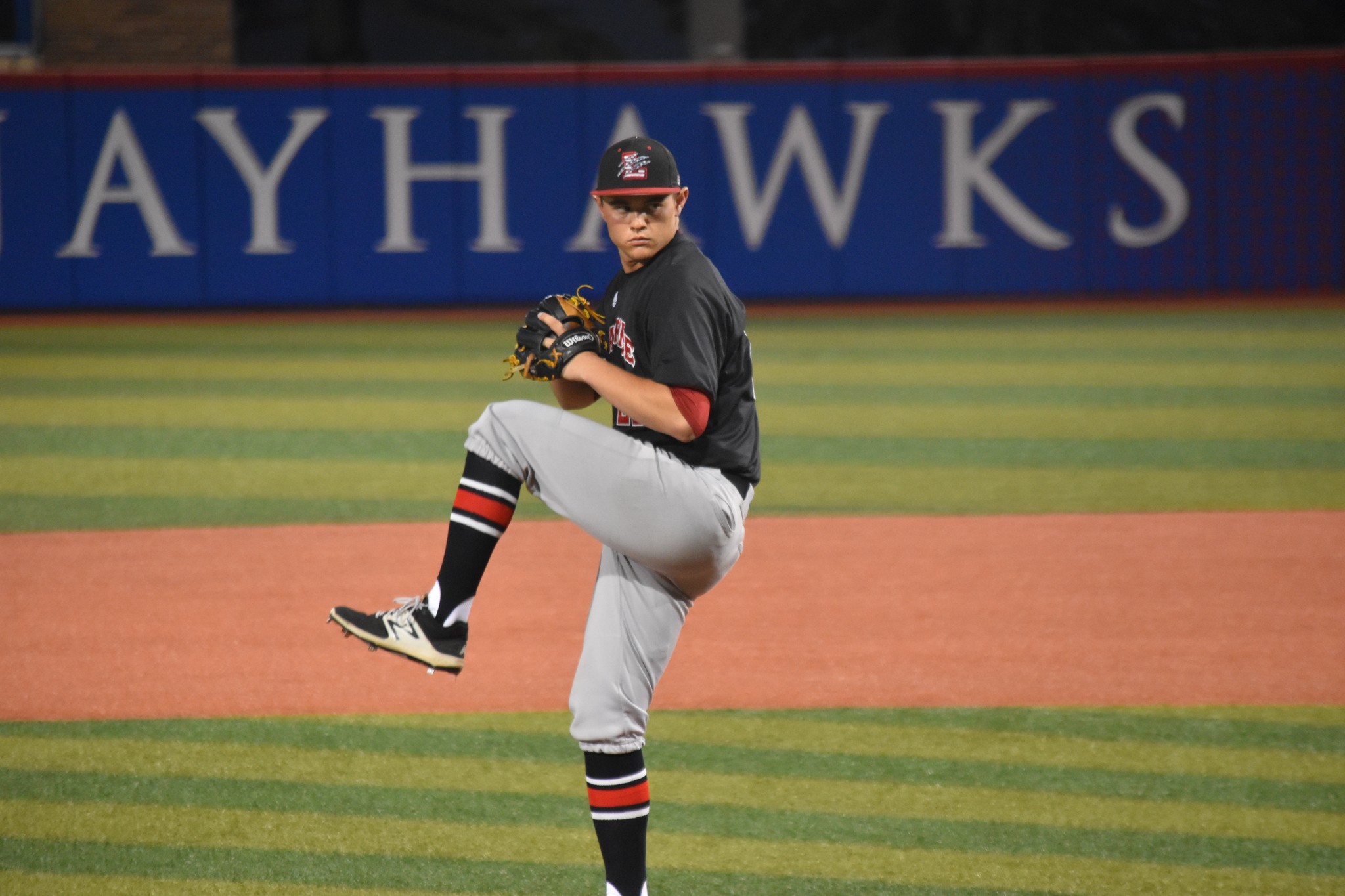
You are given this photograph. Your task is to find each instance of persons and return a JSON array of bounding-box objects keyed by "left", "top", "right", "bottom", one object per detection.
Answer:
[{"left": 326, "top": 136, "right": 762, "bottom": 896}]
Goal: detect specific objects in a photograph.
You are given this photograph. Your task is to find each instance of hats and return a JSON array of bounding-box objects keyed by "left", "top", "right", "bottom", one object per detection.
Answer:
[{"left": 590, "top": 137, "right": 681, "bottom": 197}]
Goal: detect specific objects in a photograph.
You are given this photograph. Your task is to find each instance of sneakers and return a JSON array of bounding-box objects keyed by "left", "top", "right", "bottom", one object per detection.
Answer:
[{"left": 325, "top": 595, "right": 469, "bottom": 676}]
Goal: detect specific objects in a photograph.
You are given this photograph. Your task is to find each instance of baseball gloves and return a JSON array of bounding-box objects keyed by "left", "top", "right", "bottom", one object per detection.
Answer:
[{"left": 509, "top": 287, "right": 609, "bottom": 385}]
